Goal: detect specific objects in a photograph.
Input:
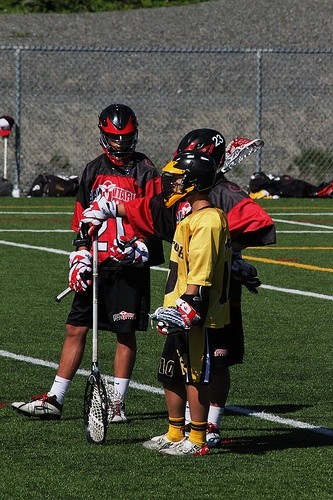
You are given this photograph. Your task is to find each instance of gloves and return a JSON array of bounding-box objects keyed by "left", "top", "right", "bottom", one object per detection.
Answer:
[
  {"left": 108, "top": 236, "right": 149, "bottom": 266},
  {"left": 237, "top": 275, "right": 262, "bottom": 294},
  {"left": 67, "top": 249, "right": 93, "bottom": 294},
  {"left": 79, "top": 199, "right": 118, "bottom": 232},
  {"left": 154, "top": 297, "right": 201, "bottom": 336}
]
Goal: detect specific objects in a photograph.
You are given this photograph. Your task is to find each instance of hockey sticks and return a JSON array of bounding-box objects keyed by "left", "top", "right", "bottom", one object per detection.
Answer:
[
  {"left": 84, "top": 231, "right": 109, "bottom": 445},
  {"left": 55, "top": 137, "right": 264, "bottom": 302},
  {"left": 147, "top": 306, "right": 185, "bottom": 328}
]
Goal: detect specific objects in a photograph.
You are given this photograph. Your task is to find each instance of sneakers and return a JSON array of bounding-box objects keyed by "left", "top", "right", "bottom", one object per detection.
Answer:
[
  {"left": 10, "top": 392, "right": 64, "bottom": 421},
  {"left": 142, "top": 432, "right": 212, "bottom": 457},
  {"left": 106, "top": 398, "right": 127, "bottom": 425},
  {"left": 182, "top": 420, "right": 222, "bottom": 447}
]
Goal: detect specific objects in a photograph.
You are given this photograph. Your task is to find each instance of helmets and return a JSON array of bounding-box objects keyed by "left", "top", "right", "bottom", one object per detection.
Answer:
[
  {"left": 172, "top": 127, "right": 227, "bottom": 171},
  {"left": 159, "top": 150, "right": 217, "bottom": 209},
  {"left": 97, "top": 103, "right": 140, "bottom": 166}
]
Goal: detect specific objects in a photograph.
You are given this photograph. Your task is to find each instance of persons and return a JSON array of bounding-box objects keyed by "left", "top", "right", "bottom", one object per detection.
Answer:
[
  {"left": 79, "top": 129, "right": 275, "bottom": 448},
  {"left": 11, "top": 105, "right": 165, "bottom": 423},
  {"left": 142, "top": 152, "right": 229, "bottom": 455}
]
